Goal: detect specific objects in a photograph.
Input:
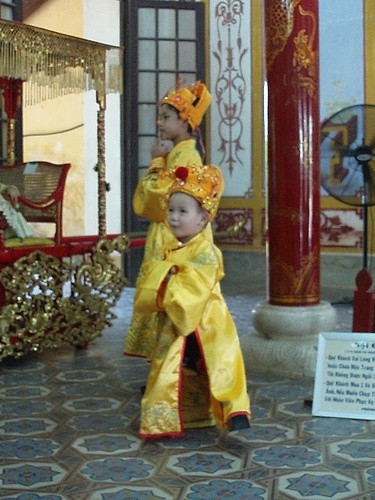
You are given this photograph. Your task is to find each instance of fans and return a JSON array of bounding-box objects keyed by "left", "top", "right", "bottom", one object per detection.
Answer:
[{"left": 318, "top": 103, "right": 375, "bottom": 305}]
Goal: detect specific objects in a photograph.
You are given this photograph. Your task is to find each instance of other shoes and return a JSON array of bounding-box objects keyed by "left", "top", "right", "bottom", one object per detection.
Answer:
[
  {"left": 227, "top": 415, "right": 251, "bottom": 432},
  {"left": 129, "top": 421, "right": 146, "bottom": 434}
]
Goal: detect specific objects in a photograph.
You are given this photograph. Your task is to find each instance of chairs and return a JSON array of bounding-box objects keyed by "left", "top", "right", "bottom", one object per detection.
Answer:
[{"left": 0, "top": 161, "right": 72, "bottom": 248}]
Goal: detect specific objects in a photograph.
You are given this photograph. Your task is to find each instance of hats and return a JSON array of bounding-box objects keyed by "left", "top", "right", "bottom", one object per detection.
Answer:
[
  {"left": 160, "top": 83, "right": 211, "bottom": 131},
  {"left": 167, "top": 164, "right": 224, "bottom": 221}
]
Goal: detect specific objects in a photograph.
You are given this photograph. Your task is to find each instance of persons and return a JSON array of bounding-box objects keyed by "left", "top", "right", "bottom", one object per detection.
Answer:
[
  {"left": 137, "top": 166, "right": 255, "bottom": 440},
  {"left": 122, "top": 82, "right": 215, "bottom": 366}
]
